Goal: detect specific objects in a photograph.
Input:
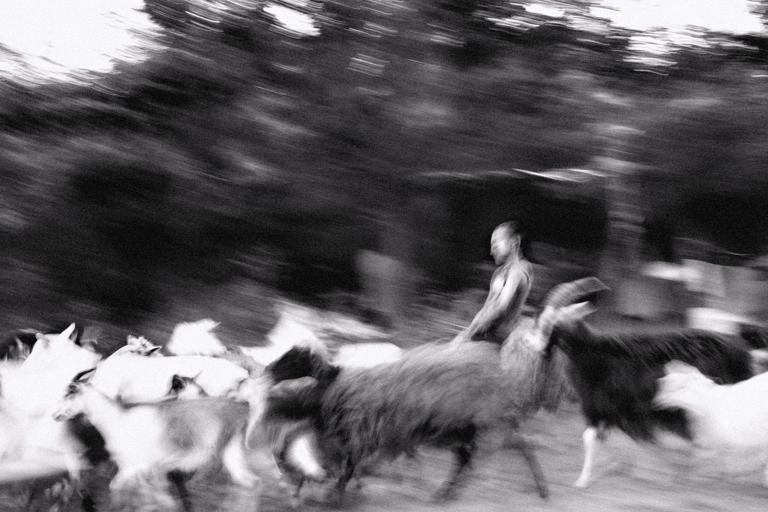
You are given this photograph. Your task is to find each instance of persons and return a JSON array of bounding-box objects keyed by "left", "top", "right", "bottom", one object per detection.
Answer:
[{"left": 456, "top": 218, "right": 533, "bottom": 344}]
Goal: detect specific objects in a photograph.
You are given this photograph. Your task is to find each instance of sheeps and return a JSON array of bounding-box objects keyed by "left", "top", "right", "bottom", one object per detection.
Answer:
[
  {"left": 1, "top": 299, "right": 401, "bottom": 510},
  {"left": 260, "top": 331, "right": 558, "bottom": 503},
  {"left": 535, "top": 279, "right": 762, "bottom": 489},
  {"left": 655, "top": 351, "right": 766, "bottom": 498}
]
